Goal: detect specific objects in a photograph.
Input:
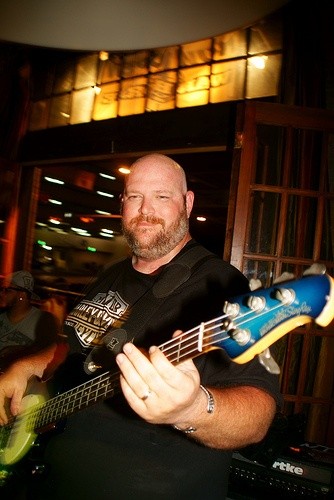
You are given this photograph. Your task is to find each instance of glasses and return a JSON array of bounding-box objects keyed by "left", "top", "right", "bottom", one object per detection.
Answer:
[{"left": 2, "top": 287, "right": 24, "bottom": 293}]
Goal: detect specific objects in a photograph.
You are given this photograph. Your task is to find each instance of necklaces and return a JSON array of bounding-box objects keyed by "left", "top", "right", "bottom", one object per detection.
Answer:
[{"left": 99, "top": 276, "right": 159, "bottom": 338}]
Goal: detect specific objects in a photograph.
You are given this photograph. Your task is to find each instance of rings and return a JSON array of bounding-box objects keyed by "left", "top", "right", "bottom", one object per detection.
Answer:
[{"left": 141, "top": 390, "right": 151, "bottom": 400}]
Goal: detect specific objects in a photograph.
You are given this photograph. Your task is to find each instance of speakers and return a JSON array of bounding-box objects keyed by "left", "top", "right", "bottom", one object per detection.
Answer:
[{"left": 226, "top": 447, "right": 334, "bottom": 500}]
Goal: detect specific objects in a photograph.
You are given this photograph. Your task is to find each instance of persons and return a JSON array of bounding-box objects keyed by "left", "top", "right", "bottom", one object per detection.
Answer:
[
  {"left": 0, "top": 153, "right": 279, "bottom": 500},
  {"left": 0, "top": 271, "right": 54, "bottom": 369}
]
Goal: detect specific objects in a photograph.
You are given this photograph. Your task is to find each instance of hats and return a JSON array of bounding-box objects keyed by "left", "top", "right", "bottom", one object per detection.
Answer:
[{"left": 4, "top": 271, "right": 33, "bottom": 292}]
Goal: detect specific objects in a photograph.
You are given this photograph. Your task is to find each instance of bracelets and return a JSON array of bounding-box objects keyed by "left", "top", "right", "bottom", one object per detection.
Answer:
[{"left": 173, "top": 385, "right": 215, "bottom": 433}]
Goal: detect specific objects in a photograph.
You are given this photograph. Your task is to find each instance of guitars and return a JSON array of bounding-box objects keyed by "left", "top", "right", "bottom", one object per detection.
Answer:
[{"left": 0, "top": 262, "right": 334, "bottom": 466}]
